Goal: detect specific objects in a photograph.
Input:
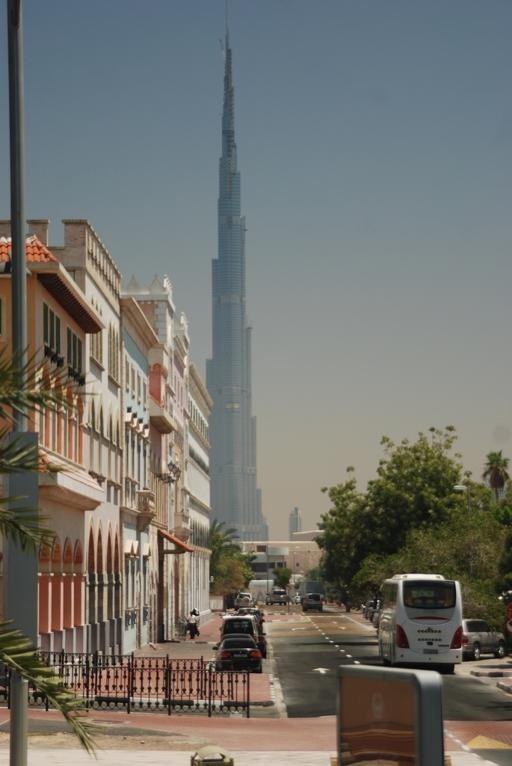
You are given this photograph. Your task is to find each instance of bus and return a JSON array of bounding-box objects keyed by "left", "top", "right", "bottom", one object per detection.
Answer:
[{"left": 377, "top": 574, "right": 462, "bottom": 674}]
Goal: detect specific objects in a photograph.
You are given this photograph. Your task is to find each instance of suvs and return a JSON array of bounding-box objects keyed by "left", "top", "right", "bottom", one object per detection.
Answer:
[
  {"left": 302, "top": 593, "right": 322, "bottom": 611},
  {"left": 461, "top": 619, "right": 506, "bottom": 660},
  {"left": 265, "top": 589, "right": 302, "bottom": 606}
]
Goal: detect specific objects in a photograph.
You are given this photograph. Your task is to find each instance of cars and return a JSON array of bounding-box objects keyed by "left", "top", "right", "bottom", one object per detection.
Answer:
[
  {"left": 211, "top": 593, "right": 267, "bottom": 673},
  {"left": 361, "top": 600, "right": 381, "bottom": 628}
]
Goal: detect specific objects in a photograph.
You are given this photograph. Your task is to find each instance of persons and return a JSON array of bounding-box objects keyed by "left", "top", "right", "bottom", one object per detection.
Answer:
[
  {"left": 184, "top": 611, "right": 198, "bottom": 639},
  {"left": 192, "top": 607, "right": 201, "bottom": 636}
]
